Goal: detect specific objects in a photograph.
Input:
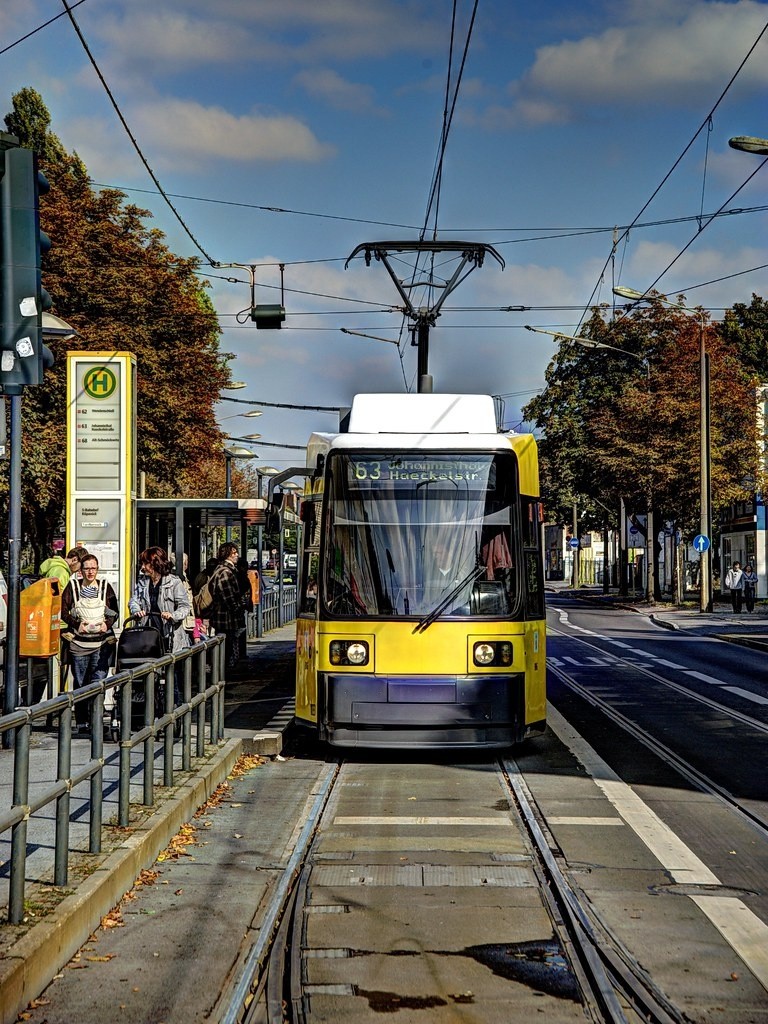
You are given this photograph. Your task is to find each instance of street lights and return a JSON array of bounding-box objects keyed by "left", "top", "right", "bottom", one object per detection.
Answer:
[
  {"left": 612, "top": 285, "right": 712, "bottom": 612},
  {"left": 257, "top": 467, "right": 281, "bottom": 638},
  {"left": 223, "top": 447, "right": 260, "bottom": 544},
  {"left": 578, "top": 338, "right": 656, "bottom": 604},
  {"left": 278, "top": 481, "right": 303, "bottom": 630}
]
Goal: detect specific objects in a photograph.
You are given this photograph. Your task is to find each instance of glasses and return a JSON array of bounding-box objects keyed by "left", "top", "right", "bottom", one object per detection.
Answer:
[{"left": 231, "top": 550, "right": 238, "bottom": 555}]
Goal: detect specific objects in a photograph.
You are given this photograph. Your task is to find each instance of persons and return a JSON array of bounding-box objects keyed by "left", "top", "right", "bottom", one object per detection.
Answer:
[
  {"left": 421, "top": 540, "right": 471, "bottom": 608},
  {"left": 61, "top": 554, "right": 120, "bottom": 733},
  {"left": 22, "top": 548, "right": 87, "bottom": 706},
  {"left": 194, "top": 542, "right": 253, "bottom": 674},
  {"left": 725, "top": 561, "right": 745, "bottom": 613},
  {"left": 128, "top": 547, "right": 196, "bottom": 715},
  {"left": 742, "top": 565, "right": 758, "bottom": 613}
]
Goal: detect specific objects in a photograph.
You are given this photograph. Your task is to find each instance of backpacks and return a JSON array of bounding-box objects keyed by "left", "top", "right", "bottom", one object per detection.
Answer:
[{"left": 194, "top": 567, "right": 231, "bottom": 610}]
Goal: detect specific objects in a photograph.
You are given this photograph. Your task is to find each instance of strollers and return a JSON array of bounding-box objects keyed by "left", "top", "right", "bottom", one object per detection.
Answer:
[{"left": 110, "top": 611, "right": 180, "bottom": 743}]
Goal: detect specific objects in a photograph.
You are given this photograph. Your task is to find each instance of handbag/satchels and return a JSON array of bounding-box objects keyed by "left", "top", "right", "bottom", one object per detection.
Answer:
[{"left": 725, "top": 570, "right": 733, "bottom": 587}]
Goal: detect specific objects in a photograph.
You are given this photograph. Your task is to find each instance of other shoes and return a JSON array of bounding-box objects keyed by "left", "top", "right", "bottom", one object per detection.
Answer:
[
  {"left": 733, "top": 611, "right": 741, "bottom": 614},
  {"left": 748, "top": 610, "right": 755, "bottom": 614},
  {"left": 78, "top": 726, "right": 91, "bottom": 735}
]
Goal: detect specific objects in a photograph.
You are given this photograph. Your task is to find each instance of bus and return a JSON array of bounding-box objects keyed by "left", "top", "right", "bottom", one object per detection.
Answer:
[{"left": 267, "top": 392, "right": 547, "bottom": 750}]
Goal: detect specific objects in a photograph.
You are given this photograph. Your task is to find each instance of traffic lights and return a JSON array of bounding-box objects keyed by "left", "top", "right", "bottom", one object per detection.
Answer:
[{"left": 0, "top": 149, "right": 55, "bottom": 397}]
